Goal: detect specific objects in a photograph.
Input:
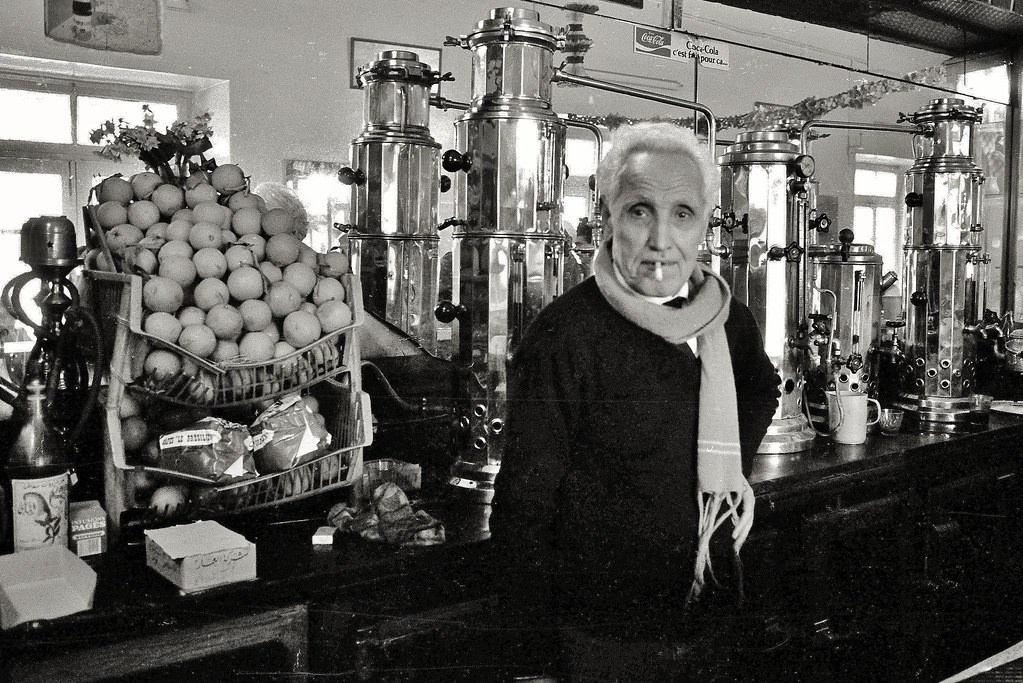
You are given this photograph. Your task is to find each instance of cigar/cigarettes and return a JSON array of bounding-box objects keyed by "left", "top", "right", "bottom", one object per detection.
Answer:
[{"left": 654, "top": 262, "right": 663, "bottom": 282}]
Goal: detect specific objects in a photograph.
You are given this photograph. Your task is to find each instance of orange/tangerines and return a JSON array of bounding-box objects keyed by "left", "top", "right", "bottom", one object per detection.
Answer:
[{"left": 87, "top": 164, "right": 352, "bottom": 517}]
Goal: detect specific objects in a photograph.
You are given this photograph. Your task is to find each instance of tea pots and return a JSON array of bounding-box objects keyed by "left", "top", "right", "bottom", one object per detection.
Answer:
[{"left": 993, "top": 328, "right": 1023, "bottom": 373}]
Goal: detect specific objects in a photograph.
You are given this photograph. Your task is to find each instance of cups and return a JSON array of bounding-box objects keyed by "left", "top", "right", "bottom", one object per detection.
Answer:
[
  {"left": 876, "top": 408, "right": 905, "bottom": 436},
  {"left": 825, "top": 390, "right": 881, "bottom": 445},
  {"left": 966, "top": 394, "right": 994, "bottom": 427}
]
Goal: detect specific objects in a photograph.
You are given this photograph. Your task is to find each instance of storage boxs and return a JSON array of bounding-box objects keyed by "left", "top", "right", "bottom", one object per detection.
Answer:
[
  {"left": 68, "top": 500, "right": 108, "bottom": 557},
  {"left": 145, "top": 536, "right": 258, "bottom": 591}
]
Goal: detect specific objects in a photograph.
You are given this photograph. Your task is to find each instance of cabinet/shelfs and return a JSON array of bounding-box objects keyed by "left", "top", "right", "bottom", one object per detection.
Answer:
[{"left": 75, "top": 270, "right": 375, "bottom": 546}]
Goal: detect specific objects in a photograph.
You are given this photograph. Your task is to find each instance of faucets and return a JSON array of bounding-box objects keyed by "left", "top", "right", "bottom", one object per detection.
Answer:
[
  {"left": 813, "top": 338, "right": 841, "bottom": 356},
  {"left": 964, "top": 309, "right": 1005, "bottom": 340}
]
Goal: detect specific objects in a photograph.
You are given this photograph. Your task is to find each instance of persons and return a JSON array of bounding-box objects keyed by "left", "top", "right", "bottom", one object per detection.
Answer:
[{"left": 485, "top": 118, "right": 781, "bottom": 683}]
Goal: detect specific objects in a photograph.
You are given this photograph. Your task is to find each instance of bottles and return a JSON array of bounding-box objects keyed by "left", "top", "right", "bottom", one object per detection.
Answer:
[{"left": 1, "top": 373, "right": 69, "bottom": 554}]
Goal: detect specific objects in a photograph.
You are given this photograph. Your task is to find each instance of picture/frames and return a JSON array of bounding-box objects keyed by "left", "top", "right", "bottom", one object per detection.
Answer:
[
  {"left": 43, "top": 0, "right": 161, "bottom": 56},
  {"left": 348, "top": 36, "right": 444, "bottom": 100}
]
